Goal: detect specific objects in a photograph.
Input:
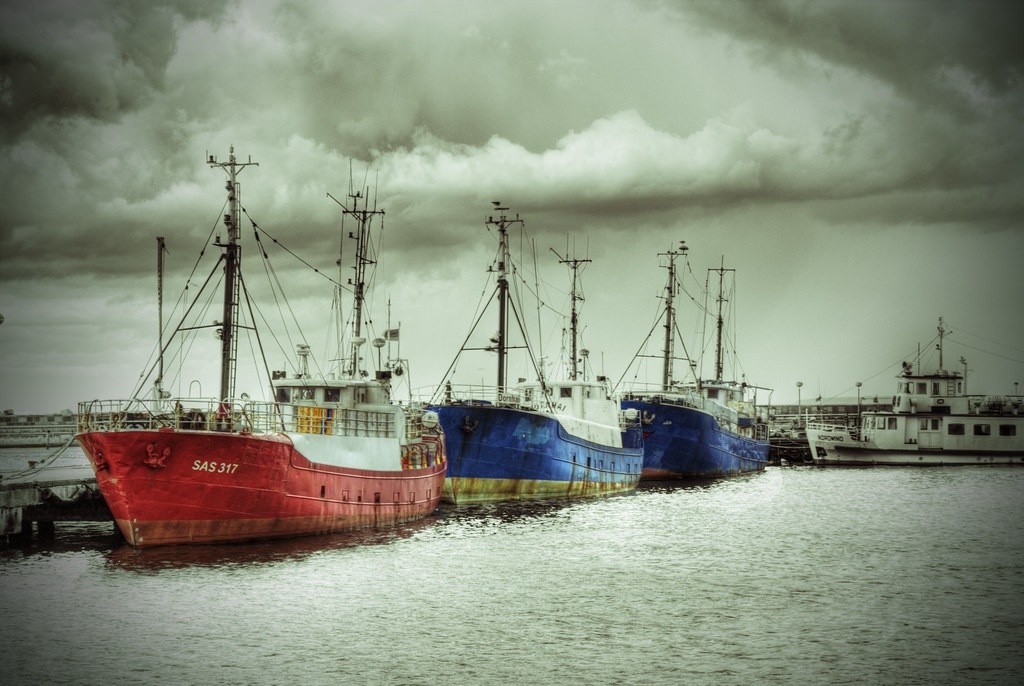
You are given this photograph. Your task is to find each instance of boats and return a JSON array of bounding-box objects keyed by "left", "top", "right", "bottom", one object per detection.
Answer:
[
  {"left": 610, "top": 233, "right": 775, "bottom": 482},
  {"left": 74, "top": 140, "right": 448, "bottom": 551},
  {"left": 804, "top": 314, "right": 1024, "bottom": 466},
  {"left": 413, "top": 195, "right": 646, "bottom": 505}
]
